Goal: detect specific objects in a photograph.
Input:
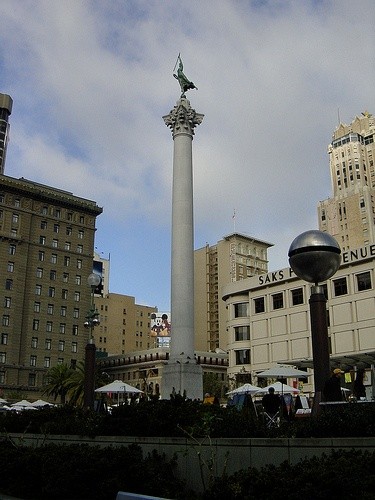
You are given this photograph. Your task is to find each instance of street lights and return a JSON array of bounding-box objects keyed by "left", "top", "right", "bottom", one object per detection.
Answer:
[{"left": 83, "top": 273, "right": 100, "bottom": 407}]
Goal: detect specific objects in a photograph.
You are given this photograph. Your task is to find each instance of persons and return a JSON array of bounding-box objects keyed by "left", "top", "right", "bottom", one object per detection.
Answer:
[
  {"left": 354, "top": 366, "right": 373, "bottom": 421},
  {"left": 323, "top": 367, "right": 351, "bottom": 428}
]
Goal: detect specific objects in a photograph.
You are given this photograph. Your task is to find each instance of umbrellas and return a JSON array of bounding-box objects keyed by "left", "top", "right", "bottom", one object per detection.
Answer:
[
  {"left": 92, "top": 378, "right": 147, "bottom": 418},
  {"left": 222, "top": 364, "right": 309, "bottom": 419},
  {"left": 0, "top": 398, "right": 57, "bottom": 414}
]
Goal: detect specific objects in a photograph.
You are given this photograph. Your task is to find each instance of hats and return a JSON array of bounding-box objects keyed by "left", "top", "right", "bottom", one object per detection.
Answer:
[{"left": 334, "top": 369, "right": 345, "bottom": 374}]
